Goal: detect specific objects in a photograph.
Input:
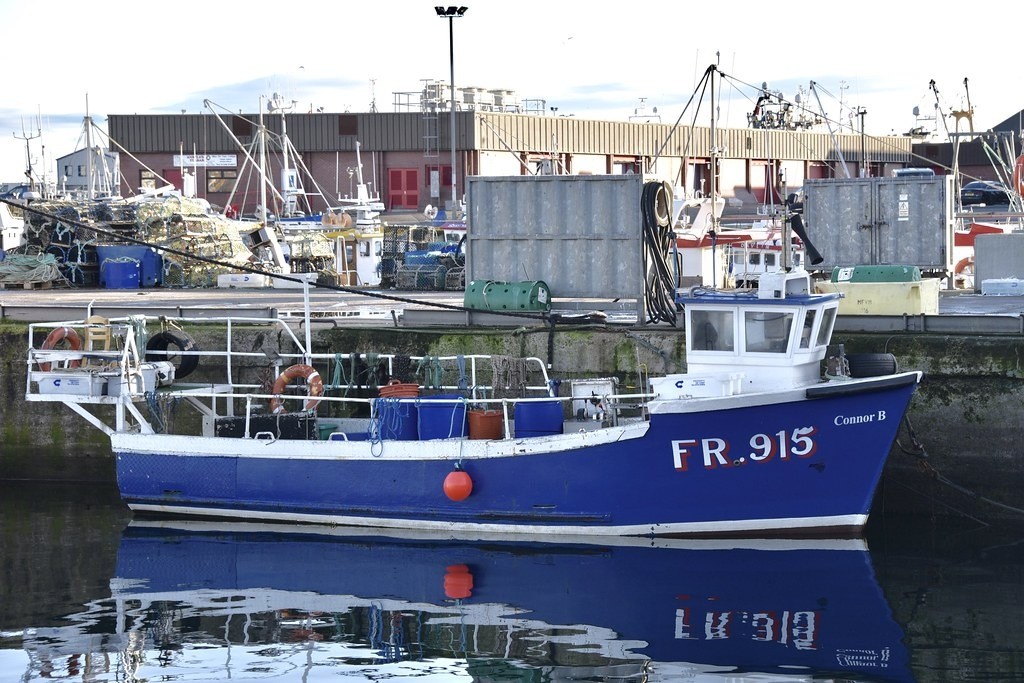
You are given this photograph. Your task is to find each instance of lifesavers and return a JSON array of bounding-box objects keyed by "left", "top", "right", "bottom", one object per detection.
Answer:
[
  {"left": 38, "top": 327, "right": 83, "bottom": 371},
  {"left": 145, "top": 330, "right": 199, "bottom": 379},
  {"left": 270, "top": 363, "right": 323, "bottom": 414}
]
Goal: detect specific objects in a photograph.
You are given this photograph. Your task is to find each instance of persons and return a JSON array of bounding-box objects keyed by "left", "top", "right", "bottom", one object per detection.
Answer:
[{"left": 694, "top": 312, "right": 718, "bottom": 350}]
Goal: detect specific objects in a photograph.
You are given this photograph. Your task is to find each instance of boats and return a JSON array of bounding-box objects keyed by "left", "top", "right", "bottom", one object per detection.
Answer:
[
  {"left": 21, "top": 536, "right": 915, "bottom": 683},
  {"left": 479, "top": 51, "right": 1024, "bottom": 288},
  {"left": 0, "top": 93, "right": 465, "bottom": 288},
  {"left": 24, "top": 267, "right": 922, "bottom": 536}
]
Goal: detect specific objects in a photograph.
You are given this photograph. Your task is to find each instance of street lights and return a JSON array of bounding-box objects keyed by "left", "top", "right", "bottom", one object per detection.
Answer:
[{"left": 435, "top": 6, "right": 469, "bottom": 219}]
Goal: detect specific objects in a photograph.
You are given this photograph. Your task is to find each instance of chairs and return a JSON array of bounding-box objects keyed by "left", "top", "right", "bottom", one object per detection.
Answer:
[{"left": 81, "top": 314, "right": 131, "bottom": 371}]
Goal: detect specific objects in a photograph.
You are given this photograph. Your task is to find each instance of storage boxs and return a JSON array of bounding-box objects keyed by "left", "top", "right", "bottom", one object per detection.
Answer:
[{"left": 34, "top": 362, "right": 177, "bottom": 398}]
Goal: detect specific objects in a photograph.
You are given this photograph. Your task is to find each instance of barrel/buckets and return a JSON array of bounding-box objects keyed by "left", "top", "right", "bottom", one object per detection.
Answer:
[
  {"left": 377, "top": 380, "right": 420, "bottom": 441},
  {"left": 468, "top": 410, "right": 504, "bottom": 440},
  {"left": 417, "top": 394, "right": 467, "bottom": 440},
  {"left": 104, "top": 259, "right": 141, "bottom": 289},
  {"left": 514, "top": 400, "right": 563, "bottom": 437}
]
